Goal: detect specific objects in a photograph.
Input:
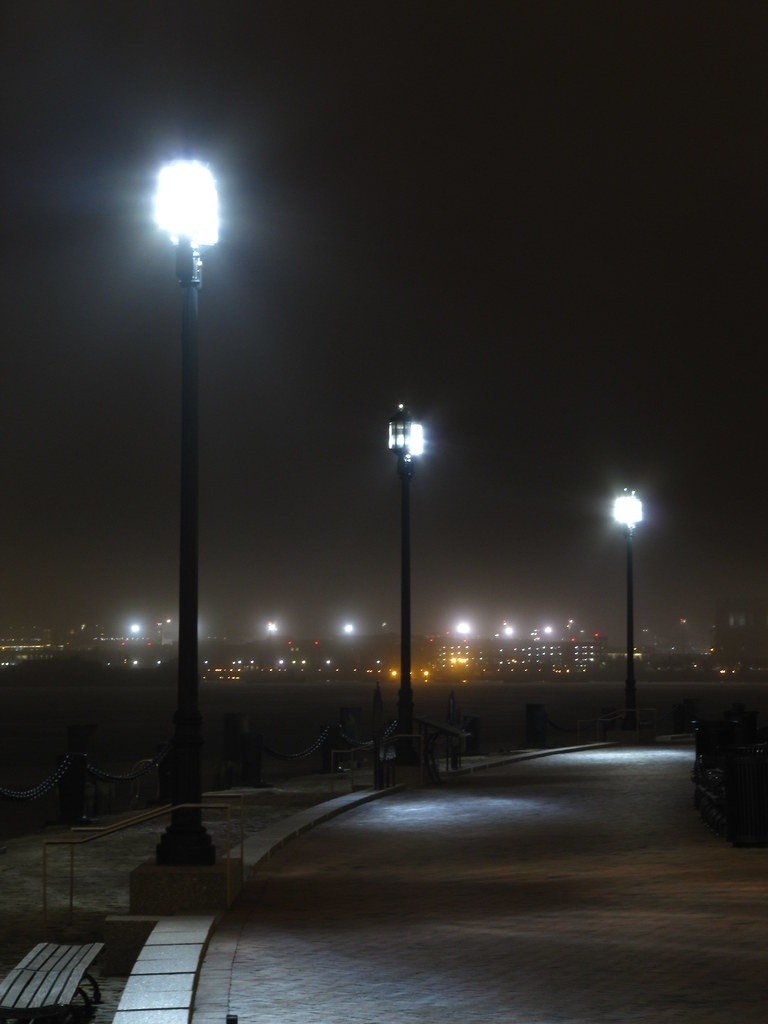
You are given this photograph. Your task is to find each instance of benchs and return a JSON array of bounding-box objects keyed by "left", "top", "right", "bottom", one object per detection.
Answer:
[{"left": 0, "top": 941, "right": 106, "bottom": 1024}]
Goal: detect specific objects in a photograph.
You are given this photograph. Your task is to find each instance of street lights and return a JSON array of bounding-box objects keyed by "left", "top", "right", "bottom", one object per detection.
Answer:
[
  {"left": 146, "top": 123, "right": 224, "bottom": 869},
  {"left": 614, "top": 483, "right": 646, "bottom": 723},
  {"left": 384, "top": 391, "right": 429, "bottom": 731}
]
